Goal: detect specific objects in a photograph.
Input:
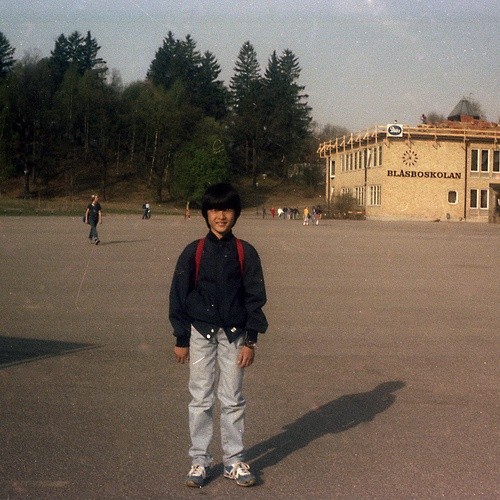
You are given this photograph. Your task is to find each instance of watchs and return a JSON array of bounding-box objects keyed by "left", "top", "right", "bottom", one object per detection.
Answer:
[{"left": 245, "top": 341, "right": 255, "bottom": 349}]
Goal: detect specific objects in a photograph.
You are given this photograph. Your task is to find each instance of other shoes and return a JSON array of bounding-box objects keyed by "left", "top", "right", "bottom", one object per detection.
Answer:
[
  {"left": 95, "top": 240, "right": 100, "bottom": 245},
  {"left": 88, "top": 237, "right": 92, "bottom": 244}
]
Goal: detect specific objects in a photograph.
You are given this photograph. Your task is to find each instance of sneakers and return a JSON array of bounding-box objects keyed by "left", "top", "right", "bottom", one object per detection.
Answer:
[
  {"left": 185, "top": 464, "right": 210, "bottom": 488},
  {"left": 223, "top": 462, "right": 256, "bottom": 486}
]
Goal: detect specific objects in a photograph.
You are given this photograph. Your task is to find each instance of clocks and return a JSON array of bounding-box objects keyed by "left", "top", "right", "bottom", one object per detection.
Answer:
[{"left": 402, "top": 150, "right": 418, "bottom": 167}]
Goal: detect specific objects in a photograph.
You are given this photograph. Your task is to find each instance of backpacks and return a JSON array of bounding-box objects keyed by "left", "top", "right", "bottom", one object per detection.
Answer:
[{"left": 83, "top": 205, "right": 99, "bottom": 224}]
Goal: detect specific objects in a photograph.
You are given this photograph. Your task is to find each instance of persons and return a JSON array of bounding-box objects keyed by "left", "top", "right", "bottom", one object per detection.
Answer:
[
  {"left": 277, "top": 206, "right": 300, "bottom": 220},
  {"left": 184, "top": 201, "right": 191, "bottom": 220},
  {"left": 168, "top": 183, "right": 269, "bottom": 488},
  {"left": 302, "top": 205, "right": 323, "bottom": 226},
  {"left": 271, "top": 207, "right": 275, "bottom": 218},
  {"left": 262, "top": 206, "right": 266, "bottom": 219},
  {"left": 142, "top": 200, "right": 151, "bottom": 220},
  {"left": 85, "top": 193, "right": 102, "bottom": 245}
]
